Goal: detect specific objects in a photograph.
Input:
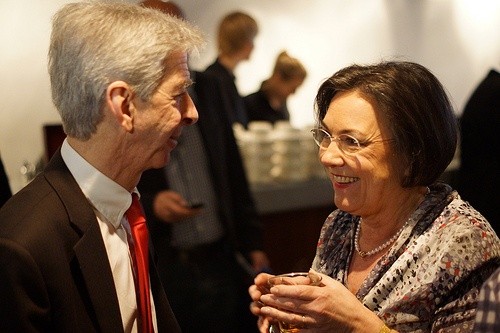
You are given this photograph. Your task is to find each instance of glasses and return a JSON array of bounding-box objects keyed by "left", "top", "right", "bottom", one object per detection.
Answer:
[{"left": 311, "top": 129, "right": 398, "bottom": 156}]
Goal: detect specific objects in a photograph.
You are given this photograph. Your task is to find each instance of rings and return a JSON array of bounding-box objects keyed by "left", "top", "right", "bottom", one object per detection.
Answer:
[{"left": 302, "top": 316, "right": 305, "bottom": 328}]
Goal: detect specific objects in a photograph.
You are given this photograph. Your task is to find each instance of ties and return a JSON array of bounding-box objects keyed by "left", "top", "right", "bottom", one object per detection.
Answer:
[{"left": 124, "top": 193, "right": 154, "bottom": 333}]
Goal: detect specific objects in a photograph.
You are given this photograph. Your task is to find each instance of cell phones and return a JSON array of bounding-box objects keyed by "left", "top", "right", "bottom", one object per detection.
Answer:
[{"left": 186, "top": 202, "right": 206, "bottom": 209}]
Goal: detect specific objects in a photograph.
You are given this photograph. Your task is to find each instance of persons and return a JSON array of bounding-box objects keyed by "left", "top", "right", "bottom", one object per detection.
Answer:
[
  {"left": 249, "top": 60, "right": 500, "bottom": 333},
  {"left": 0, "top": 0, "right": 199, "bottom": 333},
  {"left": 0, "top": 1, "right": 500, "bottom": 333}
]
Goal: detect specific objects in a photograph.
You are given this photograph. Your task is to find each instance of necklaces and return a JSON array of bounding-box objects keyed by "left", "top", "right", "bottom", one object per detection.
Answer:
[{"left": 355, "top": 185, "right": 431, "bottom": 257}]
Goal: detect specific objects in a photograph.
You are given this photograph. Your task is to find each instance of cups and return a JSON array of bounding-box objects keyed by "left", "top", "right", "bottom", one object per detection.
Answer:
[{"left": 267, "top": 273, "right": 323, "bottom": 333}]
